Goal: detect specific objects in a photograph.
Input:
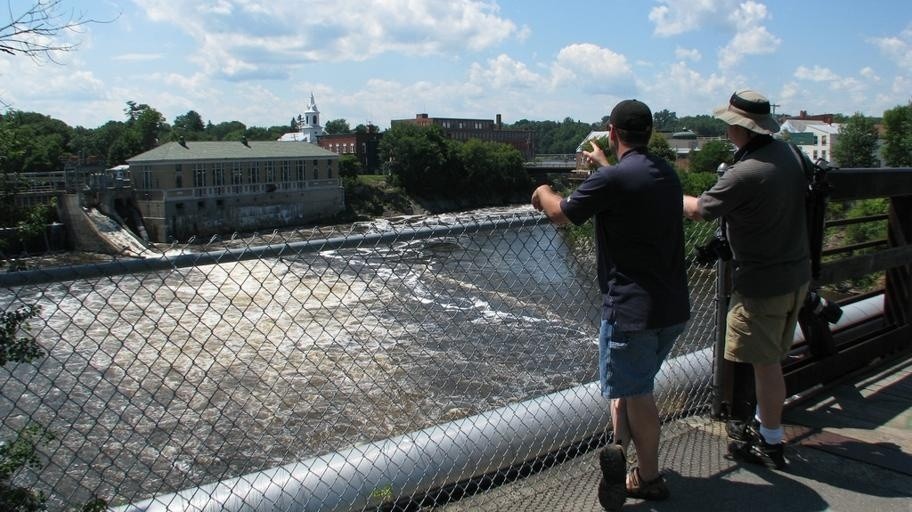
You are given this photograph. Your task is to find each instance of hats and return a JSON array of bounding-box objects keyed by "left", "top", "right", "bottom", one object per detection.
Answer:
[
  {"left": 713, "top": 87, "right": 782, "bottom": 137},
  {"left": 607, "top": 97, "right": 654, "bottom": 135}
]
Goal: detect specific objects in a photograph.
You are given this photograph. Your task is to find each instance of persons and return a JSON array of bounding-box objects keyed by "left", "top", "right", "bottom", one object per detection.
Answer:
[
  {"left": 682, "top": 88, "right": 814, "bottom": 470},
  {"left": 531, "top": 97, "right": 692, "bottom": 512}
]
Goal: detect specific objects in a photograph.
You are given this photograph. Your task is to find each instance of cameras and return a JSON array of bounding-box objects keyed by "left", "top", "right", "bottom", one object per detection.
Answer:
[
  {"left": 803, "top": 289, "right": 844, "bottom": 325},
  {"left": 693, "top": 233, "right": 733, "bottom": 265}
]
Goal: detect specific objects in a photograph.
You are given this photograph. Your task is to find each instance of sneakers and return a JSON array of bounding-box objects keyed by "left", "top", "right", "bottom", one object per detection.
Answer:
[
  {"left": 594, "top": 442, "right": 629, "bottom": 512},
  {"left": 622, "top": 464, "right": 671, "bottom": 501},
  {"left": 729, "top": 441, "right": 786, "bottom": 469},
  {"left": 725, "top": 412, "right": 763, "bottom": 445}
]
